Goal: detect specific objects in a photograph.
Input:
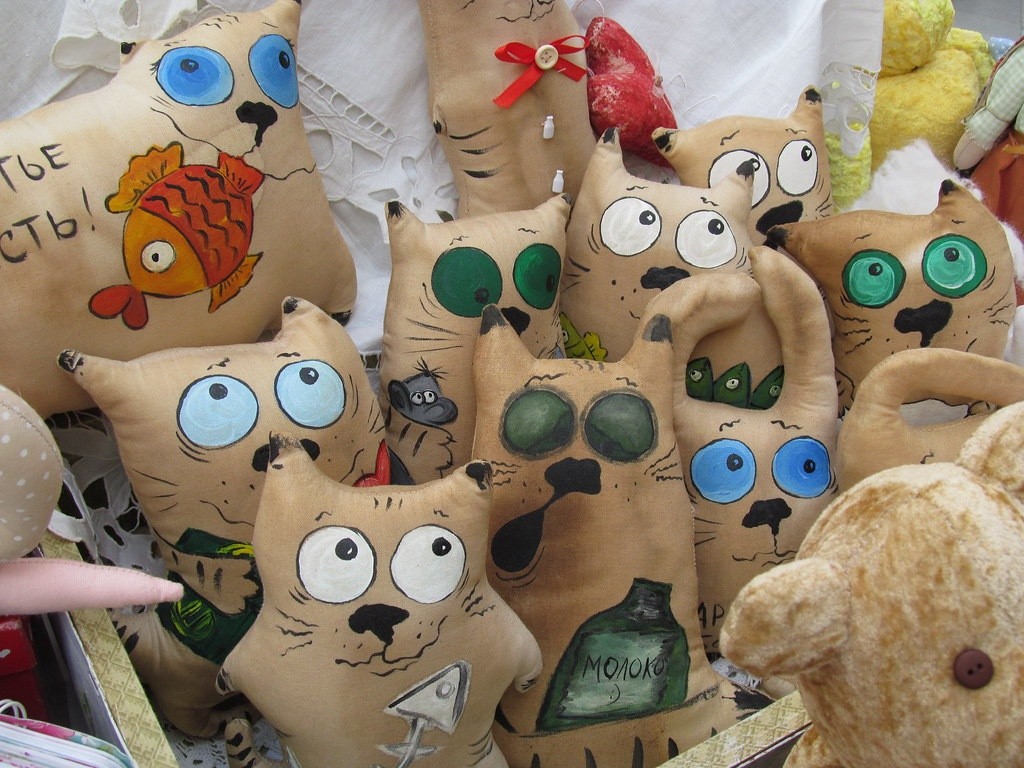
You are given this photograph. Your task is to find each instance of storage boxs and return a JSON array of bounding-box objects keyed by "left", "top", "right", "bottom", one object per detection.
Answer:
[{"left": 35, "top": 514, "right": 813, "bottom": 768}]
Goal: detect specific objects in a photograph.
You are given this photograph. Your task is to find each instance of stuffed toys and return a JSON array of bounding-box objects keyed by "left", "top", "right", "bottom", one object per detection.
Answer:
[
  {"left": 952, "top": 38, "right": 1024, "bottom": 307},
  {"left": 3, "top": 0, "right": 1023, "bottom": 766},
  {"left": 720, "top": 400, "right": 1023, "bottom": 768}
]
